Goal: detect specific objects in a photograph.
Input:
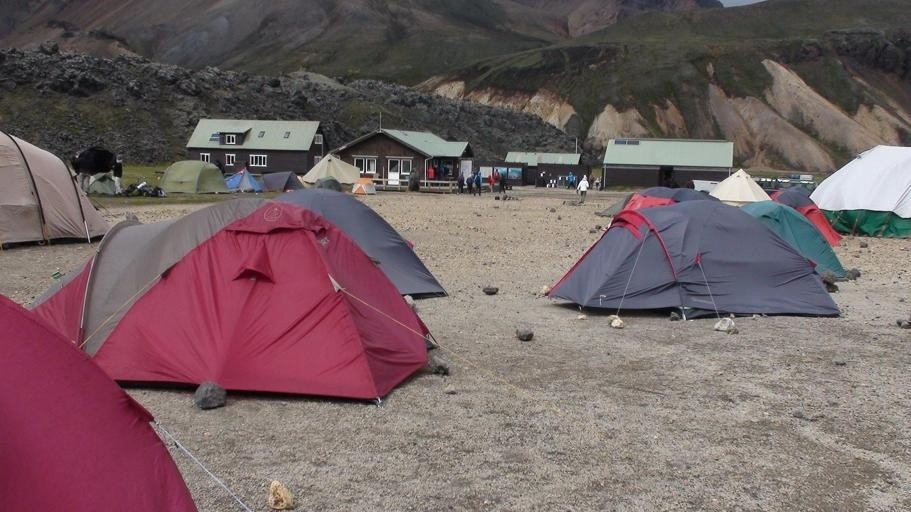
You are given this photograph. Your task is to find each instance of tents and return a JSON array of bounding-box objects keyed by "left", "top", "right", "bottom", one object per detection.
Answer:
[
  {"left": 811, "top": 143, "right": 911, "bottom": 238},
  {"left": 709, "top": 168, "right": 773, "bottom": 208},
  {"left": 302, "top": 151, "right": 362, "bottom": 185},
  {"left": 89, "top": 172, "right": 117, "bottom": 197},
  {"left": 544, "top": 195, "right": 842, "bottom": 319},
  {"left": 770, "top": 187, "right": 845, "bottom": 248},
  {"left": 741, "top": 200, "right": 850, "bottom": 283},
  {"left": 312, "top": 175, "right": 343, "bottom": 192},
  {"left": 225, "top": 166, "right": 263, "bottom": 193},
  {"left": 595, "top": 183, "right": 723, "bottom": 218},
  {"left": 609, "top": 192, "right": 677, "bottom": 223},
  {"left": 262, "top": 171, "right": 305, "bottom": 192},
  {"left": 22, "top": 194, "right": 436, "bottom": 405},
  {"left": 351, "top": 178, "right": 377, "bottom": 197},
  {"left": 161, "top": 158, "right": 232, "bottom": 195},
  {"left": 1, "top": 296, "right": 254, "bottom": 511},
  {"left": 276, "top": 183, "right": 449, "bottom": 302},
  {"left": 0, "top": 129, "right": 109, "bottom": 248}
]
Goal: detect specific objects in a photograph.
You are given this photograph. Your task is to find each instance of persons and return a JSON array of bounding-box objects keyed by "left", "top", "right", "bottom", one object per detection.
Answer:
[
  {"left": 568, "top": 171, "right": 577, "bottom": 189},
  {"left": 576, "top": 174, "right": 590, "bottom": 205},
  {"left": 112, "top": 154, "right": 125, "bottom": 196},
  {"left": 427, "top": 163, "right": 509, "bottom": 197}
]
